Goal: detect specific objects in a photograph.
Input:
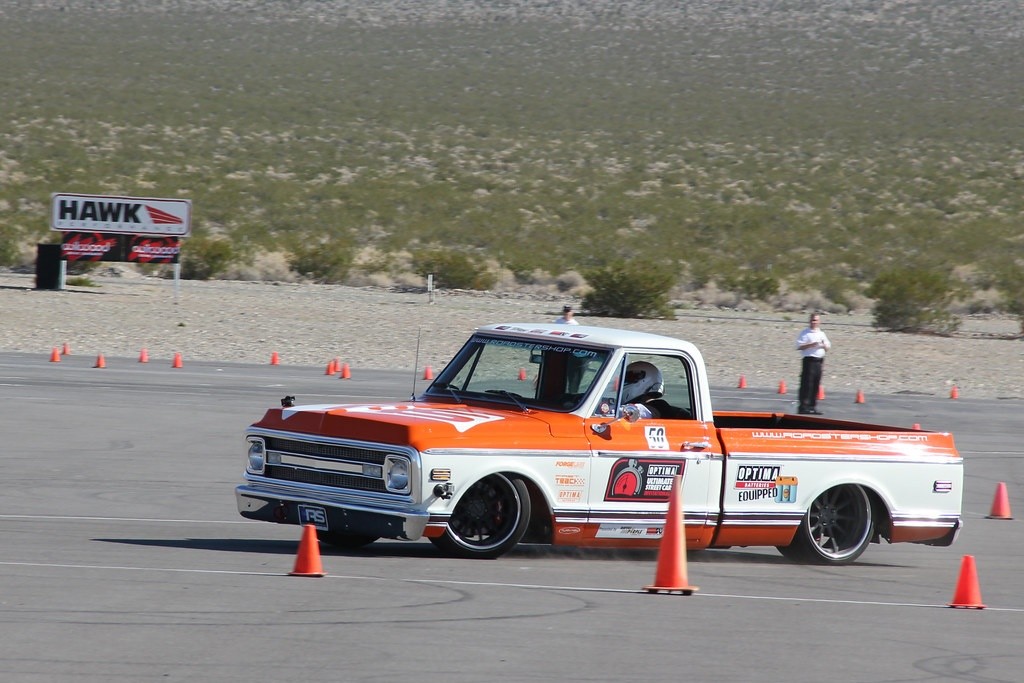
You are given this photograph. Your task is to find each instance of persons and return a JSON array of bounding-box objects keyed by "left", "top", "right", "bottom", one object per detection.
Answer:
[
  {"left": 554, "top": 306, "right": 583, "bottom": 394},
  {"left": 796, "top": 313, "right": 831, "bottom": 415},
  {"left": 593, "top": 361, "right": 682, "bottom": 420}
]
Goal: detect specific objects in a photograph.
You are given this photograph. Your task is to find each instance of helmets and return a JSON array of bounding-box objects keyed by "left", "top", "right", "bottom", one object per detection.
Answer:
[{"left": 620, "top": 361, "right": 665, "bottom": 404}]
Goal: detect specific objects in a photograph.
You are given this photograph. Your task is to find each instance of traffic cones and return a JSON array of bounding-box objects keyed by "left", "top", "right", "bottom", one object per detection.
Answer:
[
  {"left": 855, "top": 390, "right": 865, "bottom": 404},
  {"left": 172, "top": 352, "right": 183, "bottom": 368},
  {"left": 286, "top": 524, "right": 327, "bottom": 578},
  {"left": 518, "top": 368, "right": 526, "bottom": 380},
  {"left": 63, "top": 342, "right": 71, "bottom": 354},
  {"left": 139, "top": 348, "right": 148, "bottom": 363},
  {"left": 641, "top": 474, "right": 700, "bottom": 595},
  {"left": 325, "top": 360, "right": 336, "bottom": 375},
  {"left": 984, "top": 482, "right": 1014, "bottom": 520},
  {"left": 96, "top": 354, "right": 105, "bottom": 368},
  {"left": 946, "top": 554, "right": 988, "bottom": 609},
  {"left": 912, "top": 423, "right": 921, "bottom": 430},
  {"left": 423, "top": 365, "right": 434, "bottom": 380},
  {"left": 49, "top": 347, "right": 61, "bottom": 362},
  {"left": 816, "top": 385, "right": 826, "bottom": 400},
  {"left": 341, "top": 363, "right": 351, "bottom": 379},
  {"left": 271, "top": 351, "right": 280, "bottom": 365},
  {"left": 950, "top": 386, "right": 959, "bottom": 399},
  {"left": 738, "top": 375, "right": 747, "bottom": 389},
  {"left": 778, "top": 380, "right": 787, "bottom": 394},
  {"left": 333, "top": 358, "right": 341, "bottom": 372},
  {"left": 614, "top": 377, "right": 619, "bottom": 390}
]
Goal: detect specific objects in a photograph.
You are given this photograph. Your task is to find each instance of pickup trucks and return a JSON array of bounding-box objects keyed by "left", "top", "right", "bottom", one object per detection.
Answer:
[{"left": 235, "top": 322, "right": 964, "bottom": 564}]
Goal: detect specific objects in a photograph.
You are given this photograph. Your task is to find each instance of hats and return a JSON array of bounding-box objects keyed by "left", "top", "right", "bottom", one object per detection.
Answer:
[{"left": 564, "top": 306, "right": 572, "bottom": 311}]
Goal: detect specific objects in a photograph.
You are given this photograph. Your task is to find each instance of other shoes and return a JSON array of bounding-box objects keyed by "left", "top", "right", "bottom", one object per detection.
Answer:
[{"left": 799, "top": 410, "right": 825, "bottom": 414}]
